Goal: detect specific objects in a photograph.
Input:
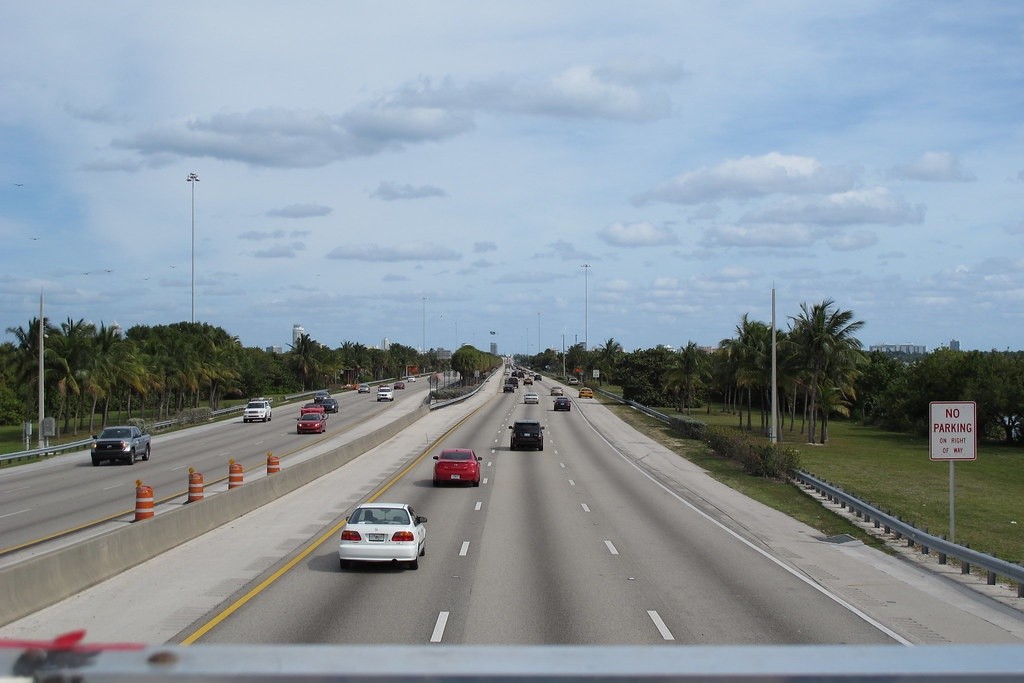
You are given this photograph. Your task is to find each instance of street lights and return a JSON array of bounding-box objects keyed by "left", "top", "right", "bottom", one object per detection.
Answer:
[
  {"left": 186, "top": 172, "right": 200, "bottom": 323},
  {"left": 580, "top": 263, "right": 591, "bottom": 351}
]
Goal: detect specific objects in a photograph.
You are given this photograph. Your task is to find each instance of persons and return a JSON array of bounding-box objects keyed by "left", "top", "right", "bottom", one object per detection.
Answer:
[{"left": 364, "top": 510, "right": 378, "bottom": 522}]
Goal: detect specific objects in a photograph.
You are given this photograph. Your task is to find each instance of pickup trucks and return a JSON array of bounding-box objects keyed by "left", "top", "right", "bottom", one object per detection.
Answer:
[{"left": 91, "top": 427, "right": 152, "bottom": 466}]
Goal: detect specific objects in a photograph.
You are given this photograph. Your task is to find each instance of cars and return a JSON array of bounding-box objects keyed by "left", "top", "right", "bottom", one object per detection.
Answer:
[
  {"left": 553, "top": 397, "right": 571, "bottom": 411},
  {"left": 432, "top": 449, "right": 482, "bottom": 487},
  {"left": 408, "top": 376, "right": 415, "bottom": 382},
  {"left": 296, "top": 391, "right": 339, "bottom": 434},
  {"left": 377, "top": 384, "right": 394, "bottom": 401},
  {"left": 337, "top": 502, "right": 428, "bottom": 570},
  {"left": 393, "top": 382, "right": 405, "bottom": 389},
  {"left": 503, "top": 370, "right": 542, "bottom": 393},
  {"left": 358, "top": 383, "right": 370, "bottom": 393},
  {"left": 568, "top": 378, "right": 579, "bottom": 384},
  {"left": 242, "top": 400, "right": 272, "bottom": 422},
  {"left": 523, "top": 392, "right": 540, "bottom": 404},
  {"left": 578, "top": 387, "right": 593, "bottom": 398},
  {"left": 550, "top": 386, "right": 564, "bottom": 396},
  {"left": 508, "top": 420, "right": 546, "bottom": 451}
]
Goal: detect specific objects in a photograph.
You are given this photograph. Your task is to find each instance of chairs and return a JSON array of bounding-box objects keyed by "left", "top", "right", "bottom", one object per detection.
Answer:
[{"left": 393, "top": 516, "right": 402, "bottom": 521}]
